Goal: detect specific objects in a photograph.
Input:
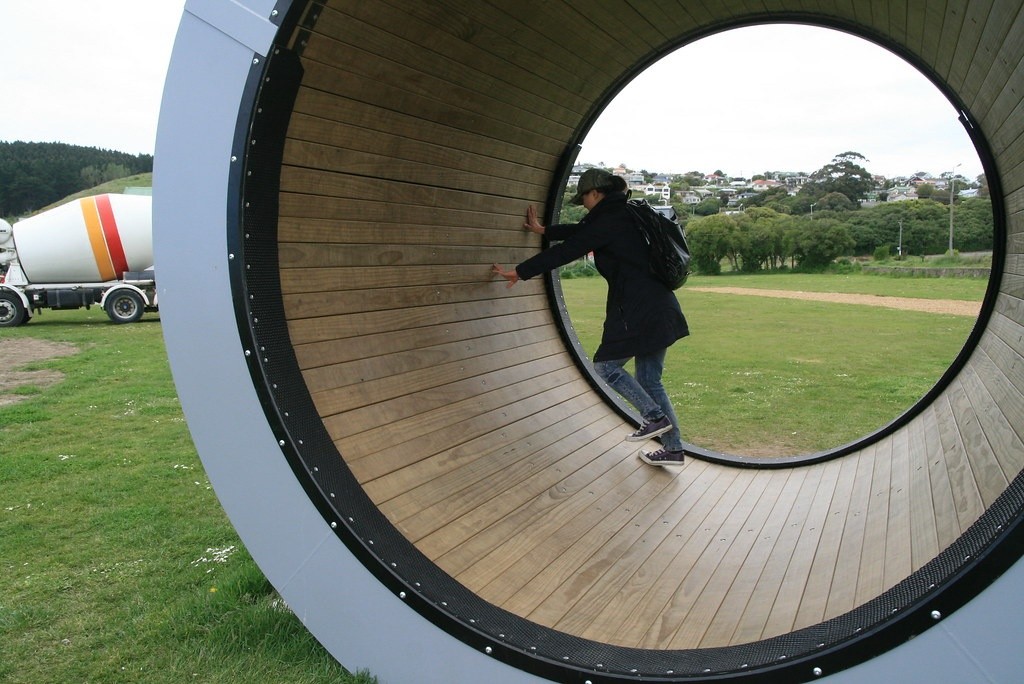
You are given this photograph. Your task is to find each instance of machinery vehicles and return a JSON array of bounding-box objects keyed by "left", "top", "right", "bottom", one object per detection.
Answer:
[{"left": 0, "top": 192, "right": 154, "bottom": 328}]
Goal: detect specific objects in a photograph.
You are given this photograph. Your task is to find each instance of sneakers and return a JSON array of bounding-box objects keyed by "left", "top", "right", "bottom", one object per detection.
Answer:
[
  {"left": 625, "top": 415, "right": 673, "bottom": 442},
  {"left": 637, "top": 445, "right": 685, "bottom": 466}
]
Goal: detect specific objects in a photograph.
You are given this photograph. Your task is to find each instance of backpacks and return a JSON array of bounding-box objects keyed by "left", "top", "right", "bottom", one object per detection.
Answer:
[{"left": 595, "top": 198, "right": 691, "bottom": 291}]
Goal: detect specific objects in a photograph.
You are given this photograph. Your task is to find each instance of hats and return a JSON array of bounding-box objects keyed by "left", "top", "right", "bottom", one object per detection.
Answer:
[{"left": 568, "top": 168, "right": 612, "bottom": 205}]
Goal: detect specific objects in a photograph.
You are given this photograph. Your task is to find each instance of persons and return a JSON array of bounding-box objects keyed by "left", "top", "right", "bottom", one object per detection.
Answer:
[{"left": 488, "top": 168, "right": 691, "bottom": 467}]
